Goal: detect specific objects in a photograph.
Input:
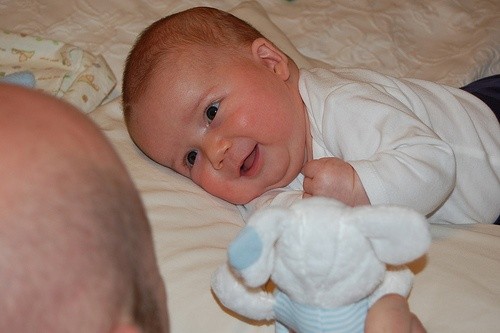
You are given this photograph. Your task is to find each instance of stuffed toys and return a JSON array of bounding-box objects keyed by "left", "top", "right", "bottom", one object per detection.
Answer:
[{"left": 210, "top": 196, "right": 430, "bottom": 333}]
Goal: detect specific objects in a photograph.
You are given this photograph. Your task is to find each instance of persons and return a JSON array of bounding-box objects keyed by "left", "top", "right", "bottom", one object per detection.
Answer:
[
  {"left": 0, "top": 83, "right": 432, "bottom": 333},
  {"left": 121, "top": 6, "right": 500, "bottom": 227}
]
